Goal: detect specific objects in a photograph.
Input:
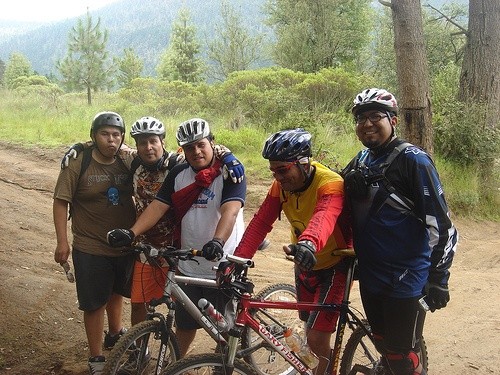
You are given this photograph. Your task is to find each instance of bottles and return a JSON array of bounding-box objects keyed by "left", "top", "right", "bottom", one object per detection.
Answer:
[
  {"left": 283, "top": 329, "right": 320, "bottom": 370},
  {"left": 198, "top": 298, "right": 229, "bottom": 332}
]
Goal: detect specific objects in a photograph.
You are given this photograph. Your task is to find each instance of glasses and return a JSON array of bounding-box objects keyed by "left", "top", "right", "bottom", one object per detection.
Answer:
[
  {"left": 270, "top": 156, "right": 304, "bottom": 175},
  {"left": 62, "top": 260, "right": 75, "bottom": 284},
  {"left": 354, "top": 114, "right": 388, "bottom": 125}
]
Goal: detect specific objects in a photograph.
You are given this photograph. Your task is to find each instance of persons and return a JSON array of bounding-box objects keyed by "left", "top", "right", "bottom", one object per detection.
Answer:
[
  {"left": 216, "top": 128, "right": 354, "bottom": 375},
  {"left": 340, "top": 88, "right": 459, "bottom": 375},
  {"left": 108, "top": 118, "right": 246, "bottom": 375},
  {"left": 61, "top": 116, "right": 245, "bottom": 375},
  {"left": 53, "top": 112, "right": 137, "bottom": 375}
]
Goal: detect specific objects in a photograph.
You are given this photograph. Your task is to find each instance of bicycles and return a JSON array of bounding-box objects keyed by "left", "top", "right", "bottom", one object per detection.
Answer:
[
  {"left": 102, "top": 239, "right": 308, "bottom": 375},
  {"left": 159, "top": 247, "right": 428, "bottom": 375}
]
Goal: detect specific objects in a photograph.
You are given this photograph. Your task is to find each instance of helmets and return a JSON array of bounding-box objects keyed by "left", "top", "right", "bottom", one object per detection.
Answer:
[
  {"left": 130, "top": 116, "right": 166, "bottom": 140},
  {"left": 262, "top": 127, "right": 313, "bottom": 161},
  {"left": 351, "top": 88, "right": 399, "bottom": 116},
  {"left": 90, "top": 111, "right": 125, "bottom": 136},
  {"left": 175, "top": 118, "right": 211, "bottom": 147}
]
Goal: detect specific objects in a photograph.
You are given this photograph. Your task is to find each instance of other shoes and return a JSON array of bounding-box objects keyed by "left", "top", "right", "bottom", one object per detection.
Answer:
[
  {"left": 104, "top": 327, "right": 138, "bottom": 351},
  {"left": 87, "top": 355, "right": 107, "bottom": 375}
]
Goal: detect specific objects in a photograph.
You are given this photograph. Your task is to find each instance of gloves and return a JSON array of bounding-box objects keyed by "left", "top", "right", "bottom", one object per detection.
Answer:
[
  {"left": 202, "top": 239, "right": 225, "bottom": 262},
  {"left": 60, "top": 142, "right": 84, "bottom": 170},
  {"left": 106, "top": 228, "right": 136, "bottom": 248},
  {"left": 221, "top": 151, "right": 246, "bottom": 183},
  {"left": 421, "top": 278, "right": 451, "bottom": 313}
]
